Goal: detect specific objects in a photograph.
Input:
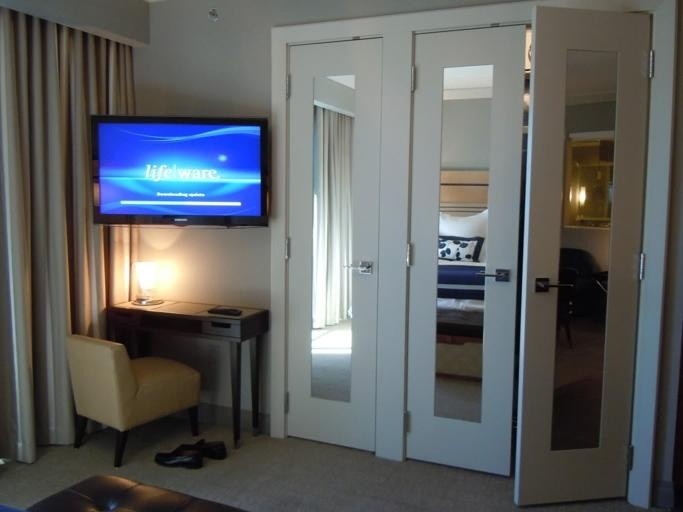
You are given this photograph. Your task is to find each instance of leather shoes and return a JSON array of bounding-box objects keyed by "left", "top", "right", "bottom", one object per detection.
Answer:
[
  {"left": 179, "top": 438, "right": 227, "bottom": 460},
  {"left": 155, "top": 444, "right": 203, "bottom": 469}
]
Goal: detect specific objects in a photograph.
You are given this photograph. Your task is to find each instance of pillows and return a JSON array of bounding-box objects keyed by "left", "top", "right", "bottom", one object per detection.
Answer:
[
  {"left": 439, "top": 209, "right": 489, "bottom": 243},
  {"left": 438, "top": 234, "right": 484, "bottom": 263}
]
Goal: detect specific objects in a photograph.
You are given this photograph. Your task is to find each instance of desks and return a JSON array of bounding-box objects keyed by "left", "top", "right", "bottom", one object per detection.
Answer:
[{"left": 107, "top": 297, "right": 271, "bottom": 449}]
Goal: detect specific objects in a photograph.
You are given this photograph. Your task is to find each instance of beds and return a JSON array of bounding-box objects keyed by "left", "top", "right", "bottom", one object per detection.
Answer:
[{"left": 436, "top": 168, "right": 491, "bottom": 381}]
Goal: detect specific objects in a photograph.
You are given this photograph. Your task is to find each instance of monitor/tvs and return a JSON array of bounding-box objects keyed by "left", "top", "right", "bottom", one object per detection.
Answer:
[{"left": 89, "top": 113, "right": 271, "bottom": 225}]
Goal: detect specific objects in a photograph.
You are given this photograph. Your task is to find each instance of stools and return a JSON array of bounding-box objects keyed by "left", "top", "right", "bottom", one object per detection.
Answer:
[{"left": 27, "top": 471, "right": 251, "bottom": 512}]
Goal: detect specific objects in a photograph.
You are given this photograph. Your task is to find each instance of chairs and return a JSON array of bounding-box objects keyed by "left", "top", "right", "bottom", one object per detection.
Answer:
[{"left": 65, "top": 334, "right": 205, "bottom": 468}]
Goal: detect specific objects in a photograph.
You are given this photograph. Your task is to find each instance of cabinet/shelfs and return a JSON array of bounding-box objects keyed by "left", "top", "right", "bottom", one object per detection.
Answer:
[{"left": 563, "top": 137, "right": 615, "bottom": 232}]
